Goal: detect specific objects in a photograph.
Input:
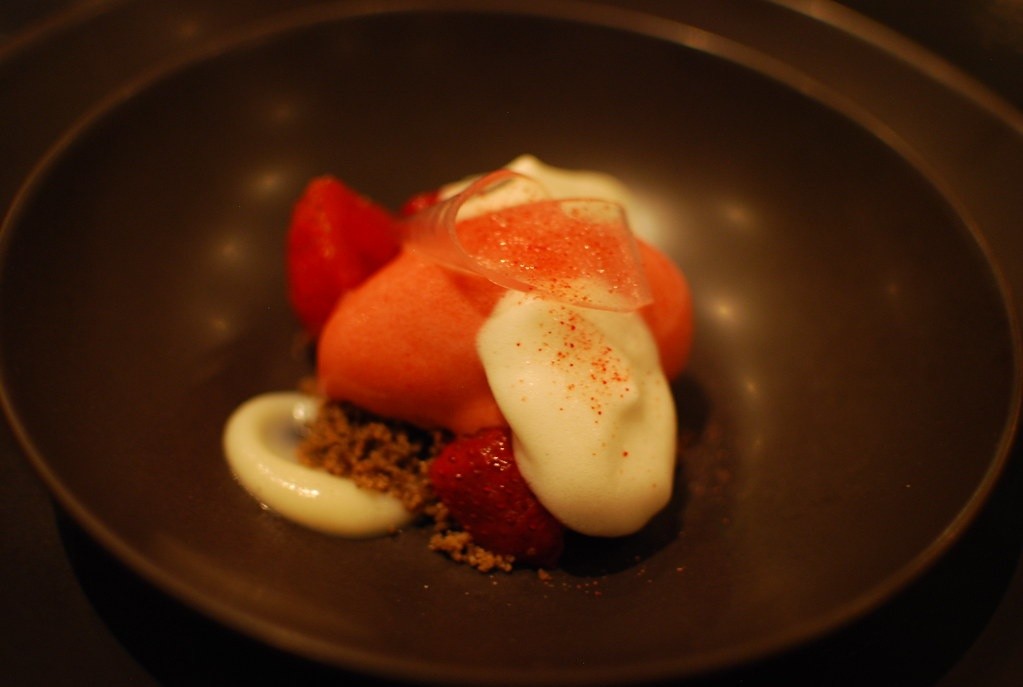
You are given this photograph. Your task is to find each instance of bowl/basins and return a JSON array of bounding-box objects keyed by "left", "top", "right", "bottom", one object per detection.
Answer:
[{"left": 0, "top": 0, "right": 1018, "bottom": 687}]
